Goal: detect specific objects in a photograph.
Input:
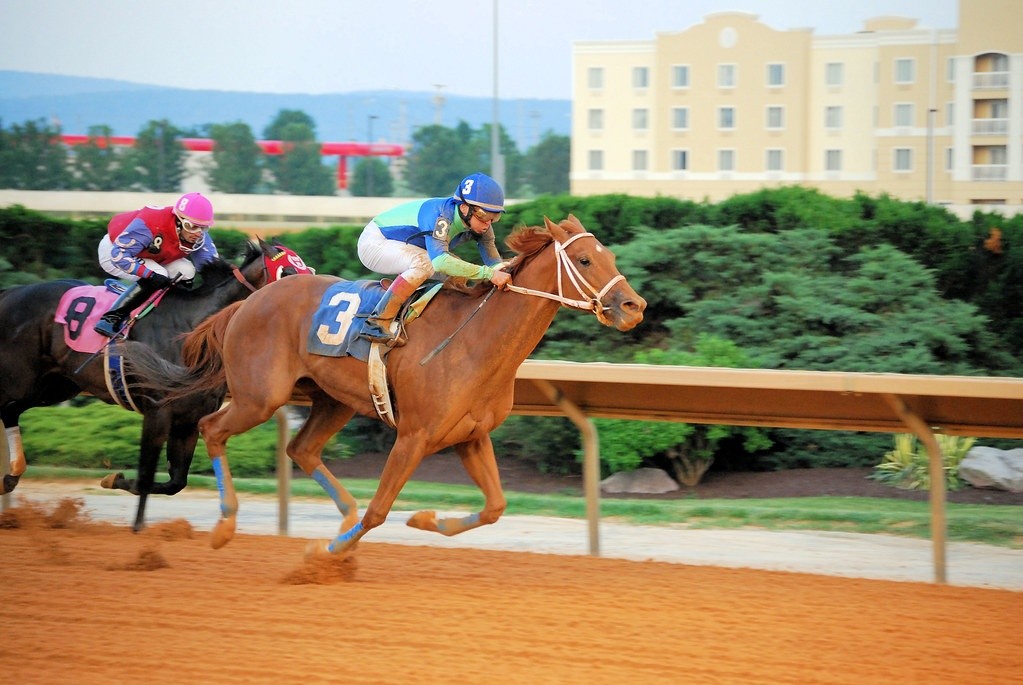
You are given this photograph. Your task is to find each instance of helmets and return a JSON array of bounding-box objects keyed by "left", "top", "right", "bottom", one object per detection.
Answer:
[
  {"left": 452, "top": 172, "right": 506, "bottom": 213},
  {"left": 174, "top": 192, "right": 216, "bottom": 226}
]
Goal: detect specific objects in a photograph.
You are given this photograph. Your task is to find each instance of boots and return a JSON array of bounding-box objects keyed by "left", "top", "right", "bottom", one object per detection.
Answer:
[
  {"left": 358, "top": 272, "right": 416, "bottom": 346},
  {"left": 94, "top": 282, "right": 149, "bottom": 340}
]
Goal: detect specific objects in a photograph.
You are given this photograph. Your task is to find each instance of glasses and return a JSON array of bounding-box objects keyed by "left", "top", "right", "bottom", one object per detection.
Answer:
[
  {"left": 182, "top": 219, "right": 208, "bottom": 235},
  {"left": 472, "top": 206, "right": 501, "bottom": 224}
]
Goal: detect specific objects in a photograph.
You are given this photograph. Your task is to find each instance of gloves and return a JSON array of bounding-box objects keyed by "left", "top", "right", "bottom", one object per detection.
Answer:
[{"left": 148, "top": 271, "right": 176, "bottom": 296}]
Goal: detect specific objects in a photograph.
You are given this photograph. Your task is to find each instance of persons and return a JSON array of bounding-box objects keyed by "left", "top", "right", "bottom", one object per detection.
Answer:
[
  {"left": 358, "top": 173, "right": 513, "bottom": 346},
  {"left": 92, "top": 193, "right": 219, "bottom": 339}
]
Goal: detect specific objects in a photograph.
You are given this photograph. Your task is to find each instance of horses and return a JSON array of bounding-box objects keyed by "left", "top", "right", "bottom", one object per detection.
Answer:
[
  {"left": 92, "top": 208, "right": 651, "bottom": 556},
  {"left": 0, "top": 231, "right": 318, "bottom": 535}
]
